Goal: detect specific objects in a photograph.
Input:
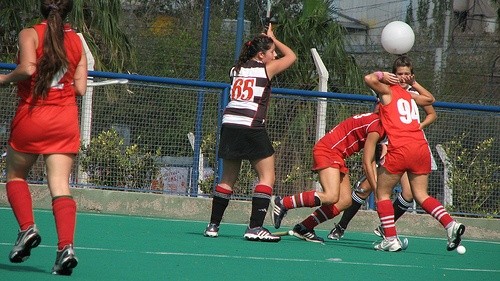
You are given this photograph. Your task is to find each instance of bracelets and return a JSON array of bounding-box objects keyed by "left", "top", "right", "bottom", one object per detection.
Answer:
[{"left": 376, "top": 72, "right": 384, "bottom": 81}]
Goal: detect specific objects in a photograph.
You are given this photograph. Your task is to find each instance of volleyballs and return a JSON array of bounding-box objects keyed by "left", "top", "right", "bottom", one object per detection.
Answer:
[{"left": 380, "top": 20, "right": 416, "bottom": 55}]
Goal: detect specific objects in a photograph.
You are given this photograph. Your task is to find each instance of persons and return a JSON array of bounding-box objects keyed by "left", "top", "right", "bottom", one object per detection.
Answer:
[
  {"left": 1, "top": 1, "right": 88, "bottom": 275},
  {"left": 270, "top": 111, "right": 386, "bottom": 244},
  {"left": 327, "top": 56, "right": 437, "bottom": 241},
  {"left": 203, "top": 21, "right": 296, "bottom": 244},
  {"left": 363, "top": 71, "right": 466, "bottom": 253}
]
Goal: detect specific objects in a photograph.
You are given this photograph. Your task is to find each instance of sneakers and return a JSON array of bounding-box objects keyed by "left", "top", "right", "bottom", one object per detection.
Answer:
[
  {"left": 445, "top": 222, "right": 466, "bottom": 251},
  {"left": 374, "top": 236, "right": 404, "bottom": 253},
  {"left": 204, "top": 223, "right": 220, "bottom": 237},
  {"left": 289, "top": 223, "right": 325, "bottom": 245},
  {"left": 374, "top": 225, "right": 385, "bottom": 236},
  {"left": 271, "top": 195, "right": 286, "bottom": 229},
  {"left": 50, "top": 244, "right": 79, "bottom": 276},
  {"left": 327, "top": 223, "right": 345, "bottom": 242},
  {"left": 8, "top": 223, "right": 43, "bottom": 262},
  {"left": 243, "top": 226, "right": 281, "bottom": 242}
]
